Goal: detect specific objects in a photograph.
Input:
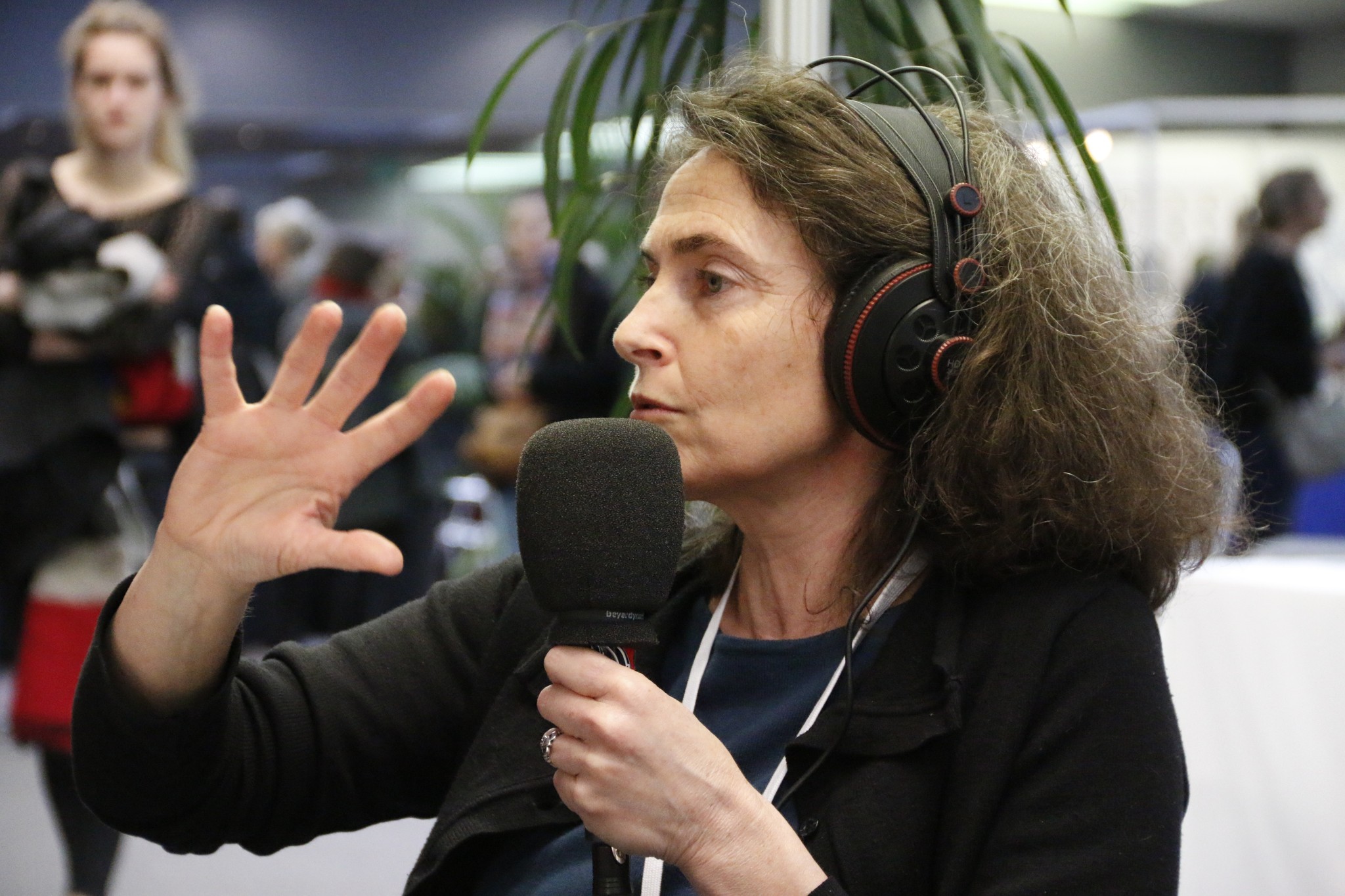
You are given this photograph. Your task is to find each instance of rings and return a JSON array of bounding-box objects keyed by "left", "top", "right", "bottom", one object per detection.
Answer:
[{"left": 541, "top": 728, "right": 565, "bottom": 769}]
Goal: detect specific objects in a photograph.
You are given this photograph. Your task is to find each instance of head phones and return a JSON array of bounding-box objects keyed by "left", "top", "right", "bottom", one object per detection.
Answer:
[{"left": 795, "top": 55, "right": 983, "bottom": 450}]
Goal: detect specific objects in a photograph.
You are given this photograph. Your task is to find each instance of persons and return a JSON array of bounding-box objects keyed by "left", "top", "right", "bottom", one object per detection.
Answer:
[
  {"left": 33, "top": 51, "right": 1261, "bottom": 895},
  {"left": 0, "top": 0, "right": 638, "bottom": 752},
  {"left": 1162, "top": 169, "right": 1329, "bottom": 551}
]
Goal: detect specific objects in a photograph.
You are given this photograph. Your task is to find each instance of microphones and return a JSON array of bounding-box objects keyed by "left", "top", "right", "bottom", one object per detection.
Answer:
[{"left": 522, "top": 414, "right": 687, "bottom": 896}]
